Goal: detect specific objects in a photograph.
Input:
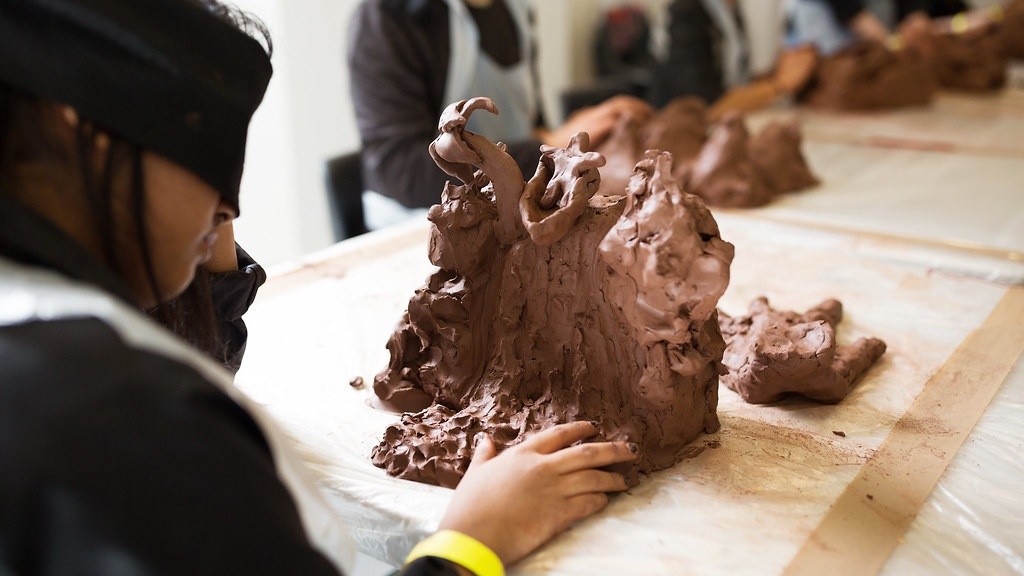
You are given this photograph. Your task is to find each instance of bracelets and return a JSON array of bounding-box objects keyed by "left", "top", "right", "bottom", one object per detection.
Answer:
[
  {"left": 952, "top": 13, "right": 969, "bottom": 33},
  {"left": 403, "top": 530, "right": 506, "bottom": 575},
  {"left": 986, "top": 4, "right": 1004, "bottom": 22},
  {"left": 886, "top": 34, "right": 905, "bottom": 51},
  {"left": 769, "top": 77, "right": 787, "bottom": 96}
]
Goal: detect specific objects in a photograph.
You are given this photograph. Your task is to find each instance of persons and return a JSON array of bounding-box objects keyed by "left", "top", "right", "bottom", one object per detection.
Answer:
[
  {"left": 649, "top": 0, "right": 1024, "bottom": 116},
  {"left": 1, "top": 0, "right": 639, "bottom": 576},
  {"left": 348, "top": 0, "right": 654, "bottom": 233}
]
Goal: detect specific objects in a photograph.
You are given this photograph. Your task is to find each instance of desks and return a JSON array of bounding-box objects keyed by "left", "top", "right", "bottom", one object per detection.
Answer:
[{"left": 233, "top": 68, "right": 1024, "bottom": 576}]
